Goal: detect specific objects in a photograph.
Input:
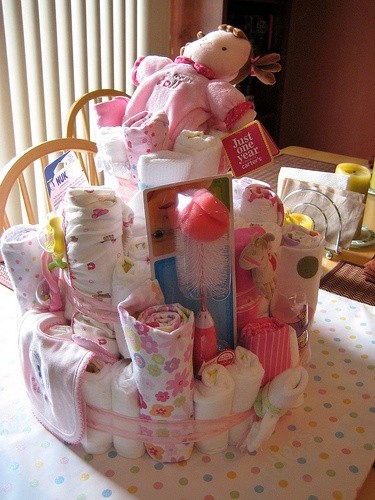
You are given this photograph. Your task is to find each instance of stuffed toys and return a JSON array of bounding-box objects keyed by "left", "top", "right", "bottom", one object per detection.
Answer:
[
  {"left": 94, "top": 25, "right": 282, "bottom": 185},
  {"left": 240, "top": 234, "right": 277, "bottom": 302}
]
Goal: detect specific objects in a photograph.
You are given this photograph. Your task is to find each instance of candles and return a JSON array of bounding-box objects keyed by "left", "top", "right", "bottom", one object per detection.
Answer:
[{"left": 334, "top": 161, "right": 372, "bottom": 240}]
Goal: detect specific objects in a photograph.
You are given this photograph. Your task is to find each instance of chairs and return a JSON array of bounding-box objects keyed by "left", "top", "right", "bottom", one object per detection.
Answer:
[
  {"left": 66, "top": 88, "right": 132, "bottom": 187},
  {"left": 0, "top": 136, "right": 105, "bottom": 266}
]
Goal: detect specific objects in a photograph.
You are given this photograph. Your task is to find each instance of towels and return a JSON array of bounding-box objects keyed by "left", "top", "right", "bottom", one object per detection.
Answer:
[{"left": 0, "top": 110, "right": 328, "bottom": 465}]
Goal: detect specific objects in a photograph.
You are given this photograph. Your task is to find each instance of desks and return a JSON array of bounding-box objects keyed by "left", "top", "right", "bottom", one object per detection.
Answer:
[{"left": 0, "top": 143, "right": 375, "bottom": 500}]
